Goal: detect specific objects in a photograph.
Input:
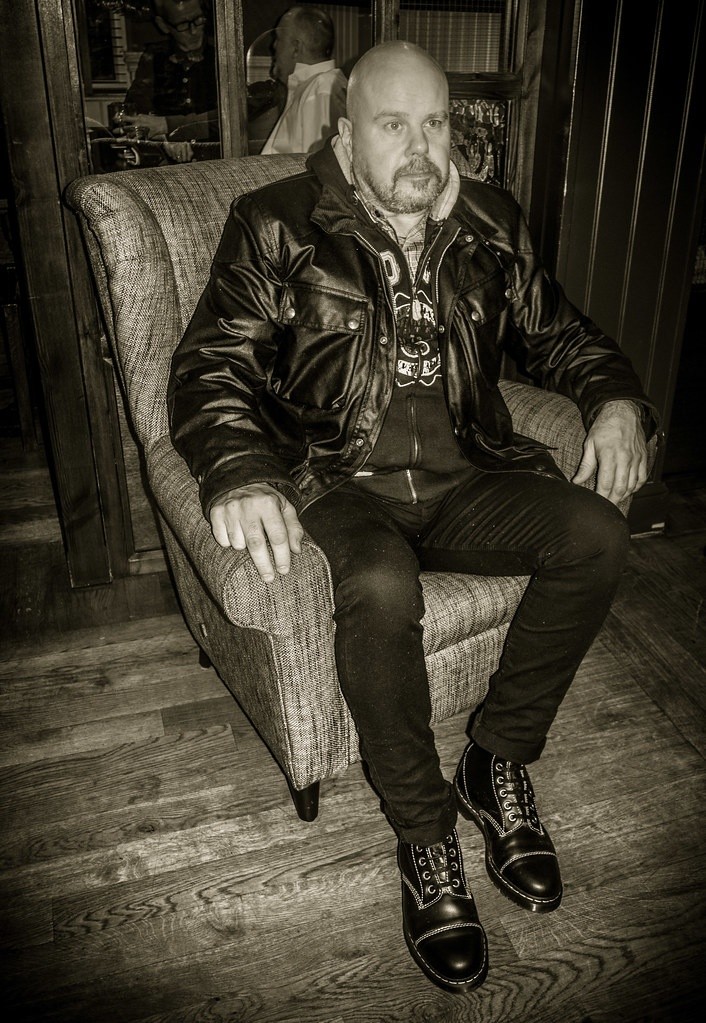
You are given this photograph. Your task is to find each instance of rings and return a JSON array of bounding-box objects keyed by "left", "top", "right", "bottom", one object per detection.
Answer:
[{"left": 176, "top": 156, "right": 181, "bottom": 159}]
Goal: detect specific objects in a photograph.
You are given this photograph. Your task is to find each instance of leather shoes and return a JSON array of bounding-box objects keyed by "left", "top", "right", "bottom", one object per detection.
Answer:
[
  {"left": 397, "top": 828, "right": 489, "bottom": 994},
  {"left": 452, "top": 739, "right": 563, "bottom": 912}
]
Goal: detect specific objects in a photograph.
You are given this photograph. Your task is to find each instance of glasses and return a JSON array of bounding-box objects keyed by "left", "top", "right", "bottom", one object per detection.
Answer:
[{"left": 164, "top": 12, "right": 206, "bottom": 32}]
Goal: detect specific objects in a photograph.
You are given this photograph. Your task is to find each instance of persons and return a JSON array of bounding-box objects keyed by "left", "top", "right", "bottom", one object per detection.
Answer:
[
  {"left": 165, "top": 41, "right": 673, "bottom": 997},
  {"left": 114, "top": 0, "right": 218, "bottom": 165},
  {"left": 260, "top": 9, "right": 351, "bottom": 156}
]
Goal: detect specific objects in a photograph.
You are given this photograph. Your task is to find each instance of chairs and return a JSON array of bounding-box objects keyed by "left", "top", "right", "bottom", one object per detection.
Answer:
[{"left": 58, "top": 152, "right": 661, "bottom": 820}]
